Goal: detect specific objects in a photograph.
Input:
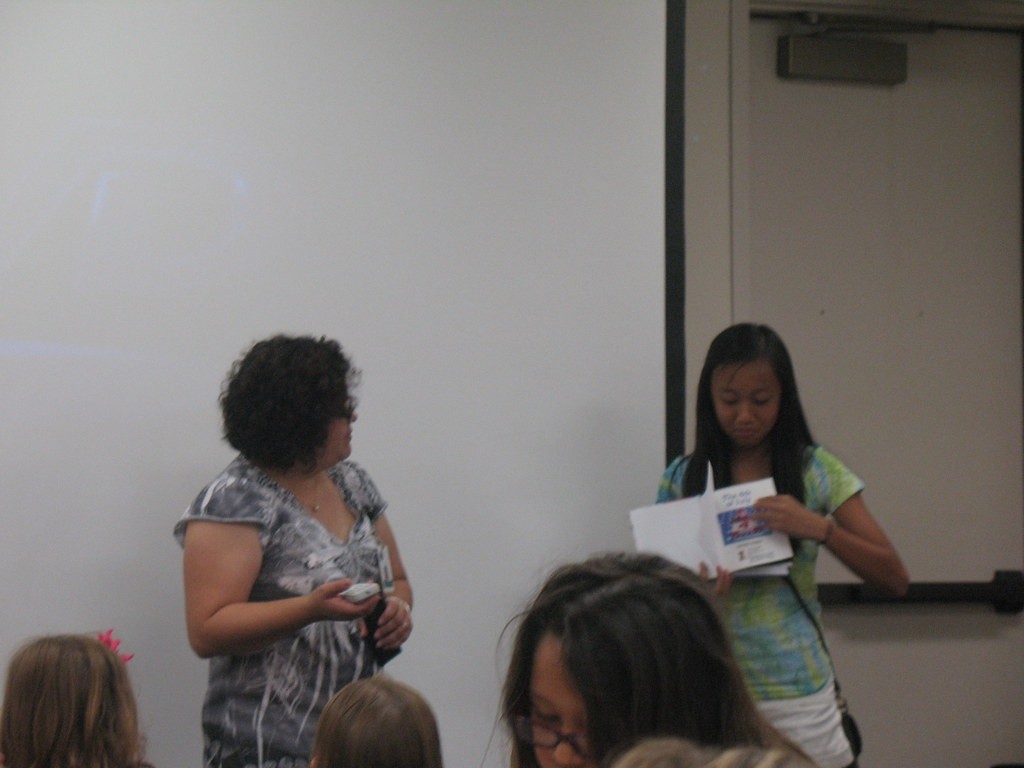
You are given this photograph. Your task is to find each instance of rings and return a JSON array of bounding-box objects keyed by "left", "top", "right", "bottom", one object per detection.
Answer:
[{"left": 402, "top": 623, "right": 409, "bottom": 631}]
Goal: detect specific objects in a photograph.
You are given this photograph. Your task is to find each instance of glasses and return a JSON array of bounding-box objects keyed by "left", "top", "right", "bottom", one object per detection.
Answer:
[{"left": 515, "top": 712, "right": 597, "bottom": 759}]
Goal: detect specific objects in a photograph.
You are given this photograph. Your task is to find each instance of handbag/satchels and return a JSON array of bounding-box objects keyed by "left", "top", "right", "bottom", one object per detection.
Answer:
[{"left": 835, "top": 696, "right": 863, "bottom": 761}]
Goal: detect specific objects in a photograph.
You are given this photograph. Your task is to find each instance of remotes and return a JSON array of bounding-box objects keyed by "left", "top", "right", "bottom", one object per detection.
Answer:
[{"left": 340, "top": 583, "right": 381, "bottom": 602}]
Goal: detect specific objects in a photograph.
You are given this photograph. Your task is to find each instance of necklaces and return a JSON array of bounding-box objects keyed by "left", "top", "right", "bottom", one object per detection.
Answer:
[{"left": 298, "top": 479, "right": 322, "bottom": 512}]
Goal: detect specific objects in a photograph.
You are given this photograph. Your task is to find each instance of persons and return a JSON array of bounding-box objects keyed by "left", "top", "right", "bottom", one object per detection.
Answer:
[
  {"left": 501, "top": 551, "right": 817, "bottom": 768},
  {"left": 655, "top": 323, "right": 908, "bottom": 768},
  {"left": 173, "top": 335, "right": 413, "bottom": 768},
  {"left": 0, "top": 634, "right": 154, "bottom": 768},
  {"left": 310, "top": 675, "right": 442, "bottom": 768}
]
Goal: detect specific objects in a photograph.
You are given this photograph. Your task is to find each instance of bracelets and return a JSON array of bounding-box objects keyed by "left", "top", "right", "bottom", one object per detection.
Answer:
[
  {"left": 386, "top": 596, "right": 410, "bottom": 614},
  {"left": 819, "top": 520, "right": 833, "bottom": 544}
]
